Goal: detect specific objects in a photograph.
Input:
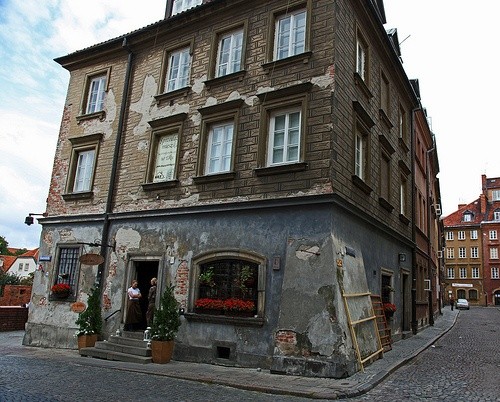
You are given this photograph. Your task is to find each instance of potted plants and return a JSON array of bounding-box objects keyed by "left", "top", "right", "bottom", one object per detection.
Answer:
[
  {"left": 147, "top": 281, "right": 182, "bottom": 363},
  {"left": 75, "top": 278, "right": 103, "bottom": 351}
]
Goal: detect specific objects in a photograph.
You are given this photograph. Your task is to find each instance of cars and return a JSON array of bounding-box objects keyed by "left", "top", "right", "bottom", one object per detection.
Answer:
[{"left": 455, "top": 299, "right": 470, "bottom": 310}]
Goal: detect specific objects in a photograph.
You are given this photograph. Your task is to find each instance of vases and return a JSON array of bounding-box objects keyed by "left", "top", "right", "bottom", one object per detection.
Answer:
[
  {"left": 220, "top": 308, "right": 233, "bottom": 315},
  {"left": 234, "top": 310, "right": 251, "bottom": 317},
  {"left": 384, "top": 309, "right": 394, "bottom": 320},
  {"left": 53, "top": 290, "right": 70, "bottom": 301}
]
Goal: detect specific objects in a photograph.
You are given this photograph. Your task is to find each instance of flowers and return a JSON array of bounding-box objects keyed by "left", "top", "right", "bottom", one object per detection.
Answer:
[
  {"left": 384, "top": 303, "right": 395, "bottom": 311},
  {"left": 195, "top": 296, "right": 255, "bottom": 312},
  {"left": 51, "top": 283, "right": 71, "bottom": 292}
]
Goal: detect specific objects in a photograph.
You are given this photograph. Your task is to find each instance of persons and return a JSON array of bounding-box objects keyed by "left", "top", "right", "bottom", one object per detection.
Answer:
[
  {"left": 146, "top": 278, "right": 157, "bottom": 327},
  {"left": 450, "top": 296, "right": 455, "bottom": 311},
  {"left": 123, "top": 280, "right": 144, "bottom": 332}
]
